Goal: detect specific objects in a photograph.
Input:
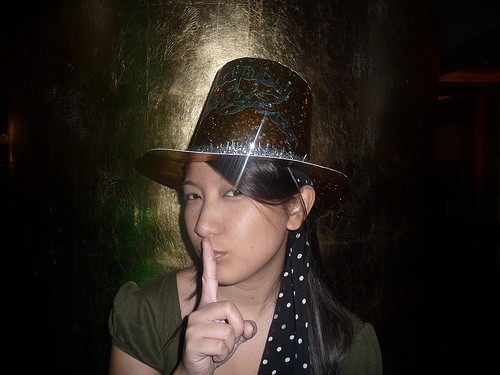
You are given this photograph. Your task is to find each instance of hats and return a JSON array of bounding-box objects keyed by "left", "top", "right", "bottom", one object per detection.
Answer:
[{"left": 142, "top": 56, "right": 347, "bottom": 218}]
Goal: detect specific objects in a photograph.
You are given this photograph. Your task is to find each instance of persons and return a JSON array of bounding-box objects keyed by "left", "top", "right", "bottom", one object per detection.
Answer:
[{"left": 108, "top": 57, "right": 386, "bottom": 375}]
